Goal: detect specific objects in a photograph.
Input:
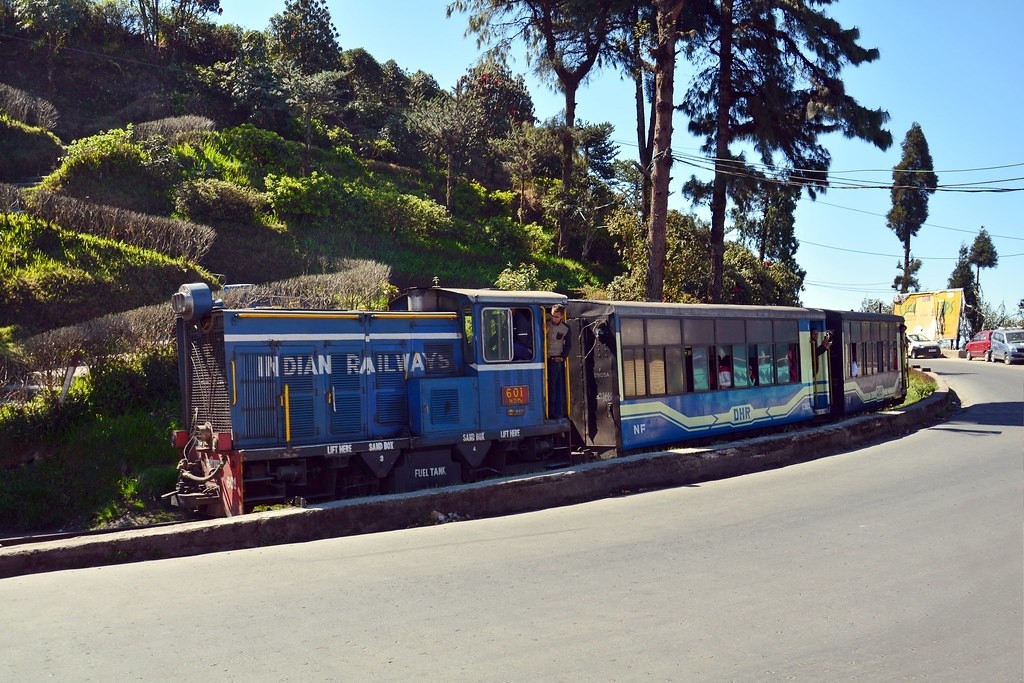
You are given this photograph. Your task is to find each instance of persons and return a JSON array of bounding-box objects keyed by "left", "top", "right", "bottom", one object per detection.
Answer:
[
  {"left": 687, "top": 351, "right": 796, "bottom": 393},
  {"left": 810, "top": 328, "right": 832, "bottom": 377},
  {"left": 485, "top": 309, "right": 533, "bottom": 360},
  {"left": 546, "top": 304, "right": 572, "bottom": 419},
  {"left": 892, "top": 348, "right": 898, "bottom": 369},
  {"left": 852, "top": 353, "right": 858, "bottom": 377}
]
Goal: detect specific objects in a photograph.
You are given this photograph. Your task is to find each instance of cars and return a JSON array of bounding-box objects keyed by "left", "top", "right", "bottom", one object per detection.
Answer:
[
  {"left": 964, "top": 328, "right": 994, "bottom": 362},
  {"left": 903, "top": 334, "right": 941, "bottom": 359}
]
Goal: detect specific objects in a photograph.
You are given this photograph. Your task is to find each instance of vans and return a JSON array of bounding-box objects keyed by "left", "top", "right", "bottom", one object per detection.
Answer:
[{"left": 991, "top": 327, "right": 1024, "bottom": 364}]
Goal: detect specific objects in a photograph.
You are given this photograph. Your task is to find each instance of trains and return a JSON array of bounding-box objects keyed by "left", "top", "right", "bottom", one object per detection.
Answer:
[{"left": 161, "top": 275, "right": 913, "bottom": 523}]
